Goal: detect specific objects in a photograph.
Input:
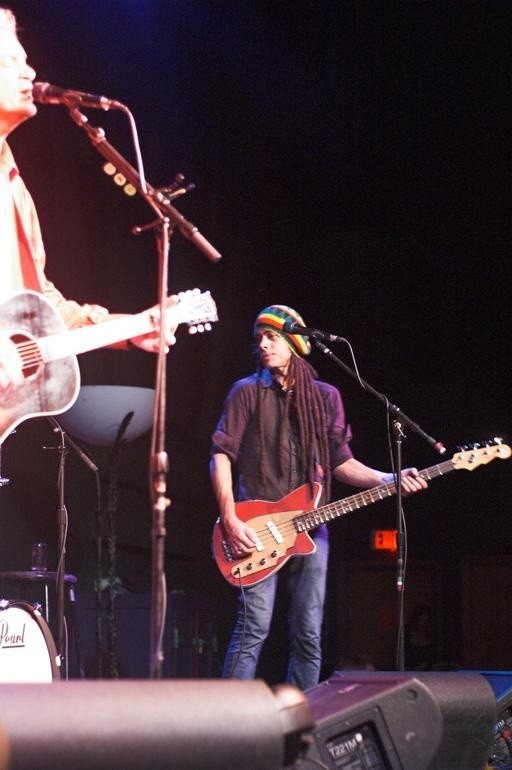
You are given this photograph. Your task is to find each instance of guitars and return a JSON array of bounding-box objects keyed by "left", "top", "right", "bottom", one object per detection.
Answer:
[
  {"left": 212, "top": 437, "right": 512, "bottom": 587},
  {"left": 1, "top": 286, "right": 219, "bottom": 443}
]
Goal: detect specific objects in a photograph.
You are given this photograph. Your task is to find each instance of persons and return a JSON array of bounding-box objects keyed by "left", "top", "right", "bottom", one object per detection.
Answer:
[
  {"left": 0, "top": 7, "right": 179, "bottom": 678},
  {"left": 210, "top": 305, "right": 429, "bottom": 687}
]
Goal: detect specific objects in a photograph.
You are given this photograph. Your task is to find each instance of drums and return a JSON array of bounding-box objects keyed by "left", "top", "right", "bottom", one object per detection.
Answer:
[{"left": 0, "top": 598, "right": 63, "bottom": 684}]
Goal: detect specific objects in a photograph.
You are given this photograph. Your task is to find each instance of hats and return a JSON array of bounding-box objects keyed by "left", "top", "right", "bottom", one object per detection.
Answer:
[{"left": 255, "top": 305, "right": 311, "bottom": 357}]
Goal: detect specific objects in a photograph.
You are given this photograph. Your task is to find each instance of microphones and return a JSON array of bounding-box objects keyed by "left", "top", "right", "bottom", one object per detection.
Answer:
[
  {"left": 282, "top": 321, "right": 339, "bottom": 343},
  {"left": 32, "top": 81, "right": 109, "bottom": 113}
]
